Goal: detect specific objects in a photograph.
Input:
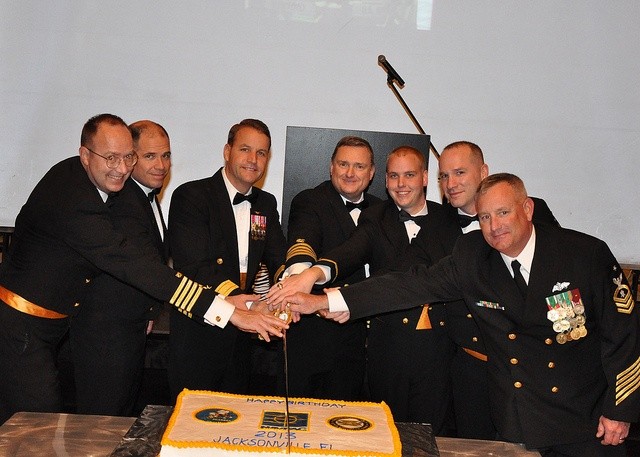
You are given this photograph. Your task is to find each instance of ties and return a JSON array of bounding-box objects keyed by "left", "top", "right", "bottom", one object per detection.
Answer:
[{"left": 512, "top": 260, "right": 528, "bottom": 299}]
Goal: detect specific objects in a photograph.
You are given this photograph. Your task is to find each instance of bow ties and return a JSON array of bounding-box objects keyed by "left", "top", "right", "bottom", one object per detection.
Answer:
[
  {"left": 346, "top": 200, "right": 369, "bottom": 212},
  {"left": 148, "top": 186, "right": 162, "bottom": 202},
  {"left": 233, "top": 192, "right": 258, "bottom": 205},
  {"left": 398, "top": 209, "right": 425, "bottom": 226},
  {"left": 458, "top": 214, "right": 480, "bottom": 228}
]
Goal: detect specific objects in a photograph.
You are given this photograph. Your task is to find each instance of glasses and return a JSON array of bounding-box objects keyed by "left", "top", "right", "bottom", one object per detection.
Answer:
[{"left": 87, "top": 147, "right": 138, "bottom": 169}]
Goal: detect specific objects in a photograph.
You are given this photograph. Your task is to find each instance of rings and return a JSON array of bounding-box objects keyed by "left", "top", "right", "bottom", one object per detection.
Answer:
[{"left": 277, "top": 283, "right": 284, "bottom": 290}]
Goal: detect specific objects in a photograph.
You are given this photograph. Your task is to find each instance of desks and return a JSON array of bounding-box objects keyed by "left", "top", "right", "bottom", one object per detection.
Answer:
[
  {"left": 0, "top": 410, "right": 542, "bottom": 457},
  {"left": 619, "top": 263, "right": 640, "bottom": 301},
  {"left": 0, "top": 226, "right": 15, "bottom": 265}
]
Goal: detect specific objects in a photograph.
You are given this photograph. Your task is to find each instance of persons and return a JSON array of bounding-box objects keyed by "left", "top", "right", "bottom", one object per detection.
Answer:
[
  {"left": 1, "top": 112, "right": 290, "bottom": 411},
  {"left": 329, "top": 141, "right": 562, "bottom": 439},
  {"left": 166, "top": 120, "right": 287, "bottom": 393},
  {"left": 280, "top": 173, "right": 639, "bottom": 455},
  {"left": 130, "top": 118, "right": 263, "bottom": 343},
  {"left": 265, "top": 146, "right": 446, "bottom": 420},
  {"left": 282, "top": 135, "right": 384, "bottom": 395}
]
